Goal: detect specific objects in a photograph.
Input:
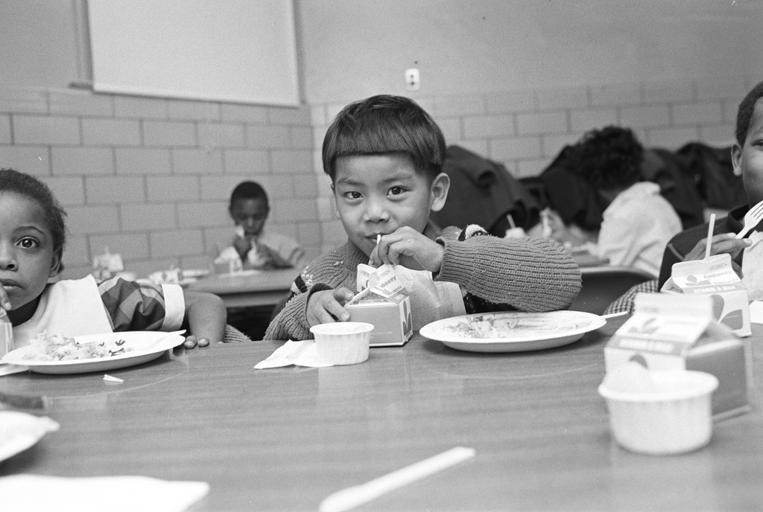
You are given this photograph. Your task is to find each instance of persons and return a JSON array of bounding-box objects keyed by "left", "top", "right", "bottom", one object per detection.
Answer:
[
  {"left": 0, "top": 167, "right": 227, "bottom": 349},
  {"left": 262, "top": 93, "right": 583, "bottom": 342},
  {"left": 227, "top": 180, "right": 305, "bottom": 270},
  {"left": 657, "top": 80, "right": 763, "bottom": 294},
  {"left": 539, "top": 124, "right": 683, "bottom": 279}
]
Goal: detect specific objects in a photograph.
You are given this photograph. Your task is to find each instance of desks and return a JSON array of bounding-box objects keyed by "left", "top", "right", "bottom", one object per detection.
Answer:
[
  {"left": 171, "top": 267, "right": 302, "bottom": 308},
  {"left": 0, "top": 314, "right": 762, "bottom": 511}
]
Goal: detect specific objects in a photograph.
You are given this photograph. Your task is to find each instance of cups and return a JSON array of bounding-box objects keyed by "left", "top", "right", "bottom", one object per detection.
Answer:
[
  {"left": 598, "top": 368, "right": 719, "bottom": 456},
  {"left": 311, "top": 322, "right": 374, "bottom": 364}
]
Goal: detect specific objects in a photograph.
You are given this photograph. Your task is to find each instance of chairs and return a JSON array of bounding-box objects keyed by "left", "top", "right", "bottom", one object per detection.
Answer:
[{"left": 567, "top": 247, "right": 656, "bottom": 314}]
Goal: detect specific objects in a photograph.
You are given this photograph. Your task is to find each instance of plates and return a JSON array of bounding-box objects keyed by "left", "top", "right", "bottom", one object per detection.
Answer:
[
  {"left": 4, "top": 330, "right": 186, "bottom": 375},
  {"left": 419, "top": 309, "right": 608, "bottom": 355}
]
